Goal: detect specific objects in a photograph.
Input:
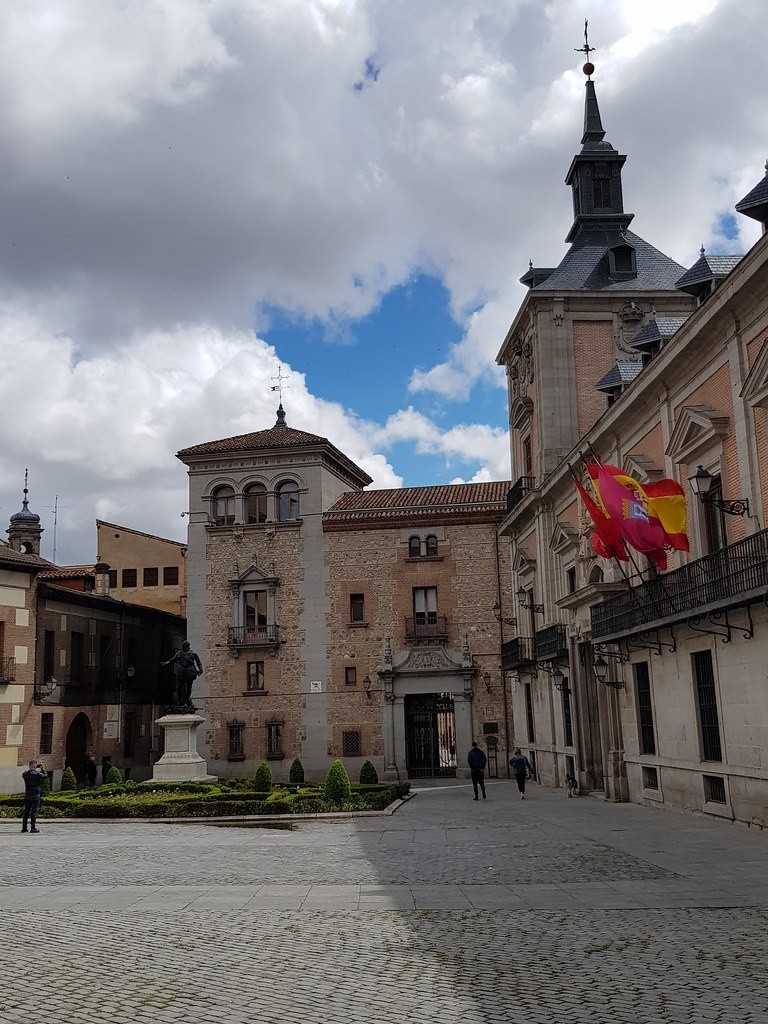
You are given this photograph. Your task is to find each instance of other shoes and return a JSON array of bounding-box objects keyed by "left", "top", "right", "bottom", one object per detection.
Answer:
[
  {"left": 521, "top": 795, "right": 524, "bottom": 800},
  {"left": 30, "top": 829, "right": 39, "bottom": 833},
  {"left": 474, "top": 798, "right": 478, "bottom": 800},
  {"left": 483, "top": 794, "right": 486, "bottom": 798},
  {"left": 21, "top": 829, "right": 28, "bottom": 832}
]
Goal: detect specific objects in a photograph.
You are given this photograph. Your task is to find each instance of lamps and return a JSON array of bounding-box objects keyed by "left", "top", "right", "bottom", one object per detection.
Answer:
[
  {"left": 687, "top": 464, "right": 751, "bottom": 520},
  {"left": 33, "top": 675, "right": 58, "bottom": 700},
  {"left": 117, "top": 664, "right": 135, "bottom": 685},
  {"left": 591, "top": 655, "right": 625, "bottom": 689},
  {"left": 492, "top": 603, "right": 517, "bottom": 627},
  {"left": 362, "top": 676, "right": 372, "bottom": 698},
  {"left": 552, "top": 666, "right": 573, "bottom": 696},
  {"left": 483, "top": 672, "right": 491, "bottom": 692},
  {"left": 514, "top": 586, "right": 544, "bottom": 614}
]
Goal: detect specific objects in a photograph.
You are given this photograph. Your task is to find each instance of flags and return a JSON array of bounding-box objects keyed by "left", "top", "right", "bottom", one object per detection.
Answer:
[{"left": 574, "top": 464, "right": 690, "bottom": 571}]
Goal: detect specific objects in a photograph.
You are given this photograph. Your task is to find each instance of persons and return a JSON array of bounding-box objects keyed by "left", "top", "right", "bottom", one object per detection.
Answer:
[
  {"left": 162, "top": 639, "right": 203, "bottom": 706},
  {"left": 467, "top": 742, "right": 487, "bottom": 801},
  {"left": 102, "top": 759, "right": 112, "bottom": 784},
  {"left": 509, "top": 748, "right": 526, "bottom": 800},
  {"left": 21, "top": 760, "right": 48, "bottom": 833},
  {"left": 86, "top": 757, "right": 97, "bottom": 787}
]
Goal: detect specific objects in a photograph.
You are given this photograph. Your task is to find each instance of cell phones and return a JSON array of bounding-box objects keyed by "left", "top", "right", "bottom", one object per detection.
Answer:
[{"left": 36, "top": 764, "right": 42, "bottom": 768}]
